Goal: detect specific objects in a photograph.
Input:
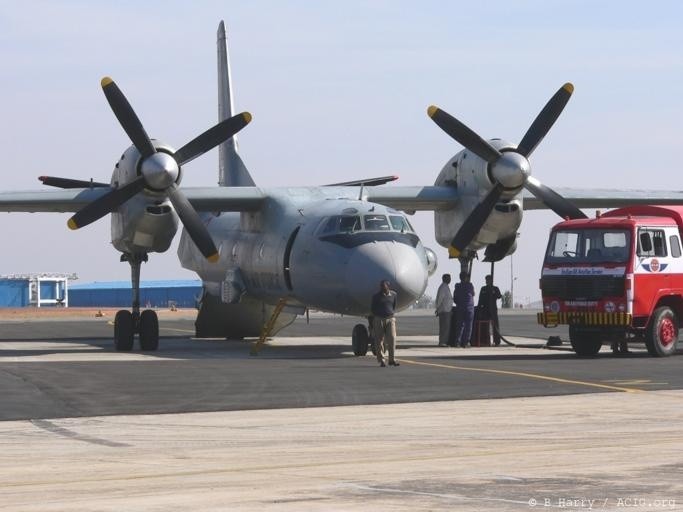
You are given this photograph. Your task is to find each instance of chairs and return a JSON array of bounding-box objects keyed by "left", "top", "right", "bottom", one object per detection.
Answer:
[{"left": 587, "top": 249, "right": 603, "bottom": 260}]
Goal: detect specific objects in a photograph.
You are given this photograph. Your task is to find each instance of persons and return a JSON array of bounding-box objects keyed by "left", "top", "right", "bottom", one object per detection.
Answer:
[
  {"left": 370, "top": 279, "right": 399, "bottom": 368},
  {"left": 434, "top": 274, "right": 453, "bottom": 347},
  {"left": 453, "top": 270, "right": 475, "bottom": 347},
  {"left": 476, "top": 274, "right": 501, "bottom": 347},
  {"left": 612, "top": 338, "right": 631, "bottom": 356}
]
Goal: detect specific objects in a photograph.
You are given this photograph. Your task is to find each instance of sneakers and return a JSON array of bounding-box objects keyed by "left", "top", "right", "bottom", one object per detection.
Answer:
[{"left": 380, "top": 361, "right": 400, "bottom": 367}]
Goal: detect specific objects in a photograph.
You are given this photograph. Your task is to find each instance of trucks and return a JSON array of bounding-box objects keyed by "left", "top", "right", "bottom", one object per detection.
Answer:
[{"left": 536, "top": 203, "right": 683, "bottom": 357}]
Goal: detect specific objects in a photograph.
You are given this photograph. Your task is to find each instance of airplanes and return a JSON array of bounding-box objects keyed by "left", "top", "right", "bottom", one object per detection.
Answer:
[{"left": 0, "top": 18, "right": 592, "bottom": 358}]
